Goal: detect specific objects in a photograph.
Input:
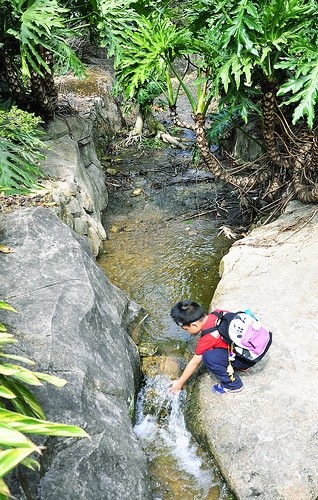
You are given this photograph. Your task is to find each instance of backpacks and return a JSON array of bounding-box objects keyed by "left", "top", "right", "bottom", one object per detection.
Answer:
[{"left": 200, "top": 312, "right": 273, "bottom": 366}]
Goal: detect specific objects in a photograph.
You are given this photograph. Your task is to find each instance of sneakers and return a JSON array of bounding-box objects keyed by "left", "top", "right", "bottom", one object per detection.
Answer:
[{"left": 213, "top": 380, "right": 244, "bottom": 394}]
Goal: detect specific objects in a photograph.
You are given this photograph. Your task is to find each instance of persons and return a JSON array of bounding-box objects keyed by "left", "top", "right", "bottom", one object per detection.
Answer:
[{"left": 167, "top": 300, "right": 272, "bottom": 396}]
